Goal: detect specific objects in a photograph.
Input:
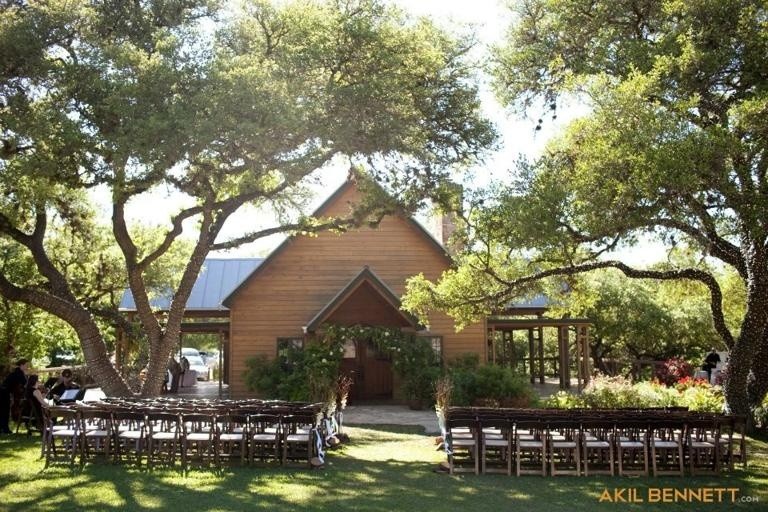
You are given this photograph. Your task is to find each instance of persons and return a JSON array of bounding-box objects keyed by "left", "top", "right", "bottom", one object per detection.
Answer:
[
  {"left": 0, "top": 358, "right": 29, "bottom": 435},
  {"left": 177, "top": 356, "right": 189, "bottom": 387},
  {"left": 702, "top": 347, "right": 720, "bottom": 383},
  {"left": 26, "top": 372, "right": 57, "bottom": 430},
  {"left": 47, "top": 368, "right": 82, "bottom": 426}
]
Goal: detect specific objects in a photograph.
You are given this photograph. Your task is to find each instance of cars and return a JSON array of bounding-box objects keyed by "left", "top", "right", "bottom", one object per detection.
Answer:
[
  {"left": 55, "top": 350, "right": 75, "bottom": 360},
  {"left": 175, "top": 347, "right": 209, "bottom": 382}
]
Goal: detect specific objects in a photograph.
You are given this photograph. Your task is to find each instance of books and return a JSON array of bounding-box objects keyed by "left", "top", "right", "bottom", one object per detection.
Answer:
[{"left": 44, "top": 376, "right": 60, "bottom": 390}]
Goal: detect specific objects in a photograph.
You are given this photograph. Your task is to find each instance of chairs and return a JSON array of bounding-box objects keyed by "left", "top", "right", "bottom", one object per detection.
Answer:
[
  {"left": 443, "top": 405, "right": 749, "bottom": 481},
  {"left": 15, "top": 399, "right": 40, "bottom": 440},
  {"left": 41, "top": 396, "right": 328, "bottom": 475}
]
